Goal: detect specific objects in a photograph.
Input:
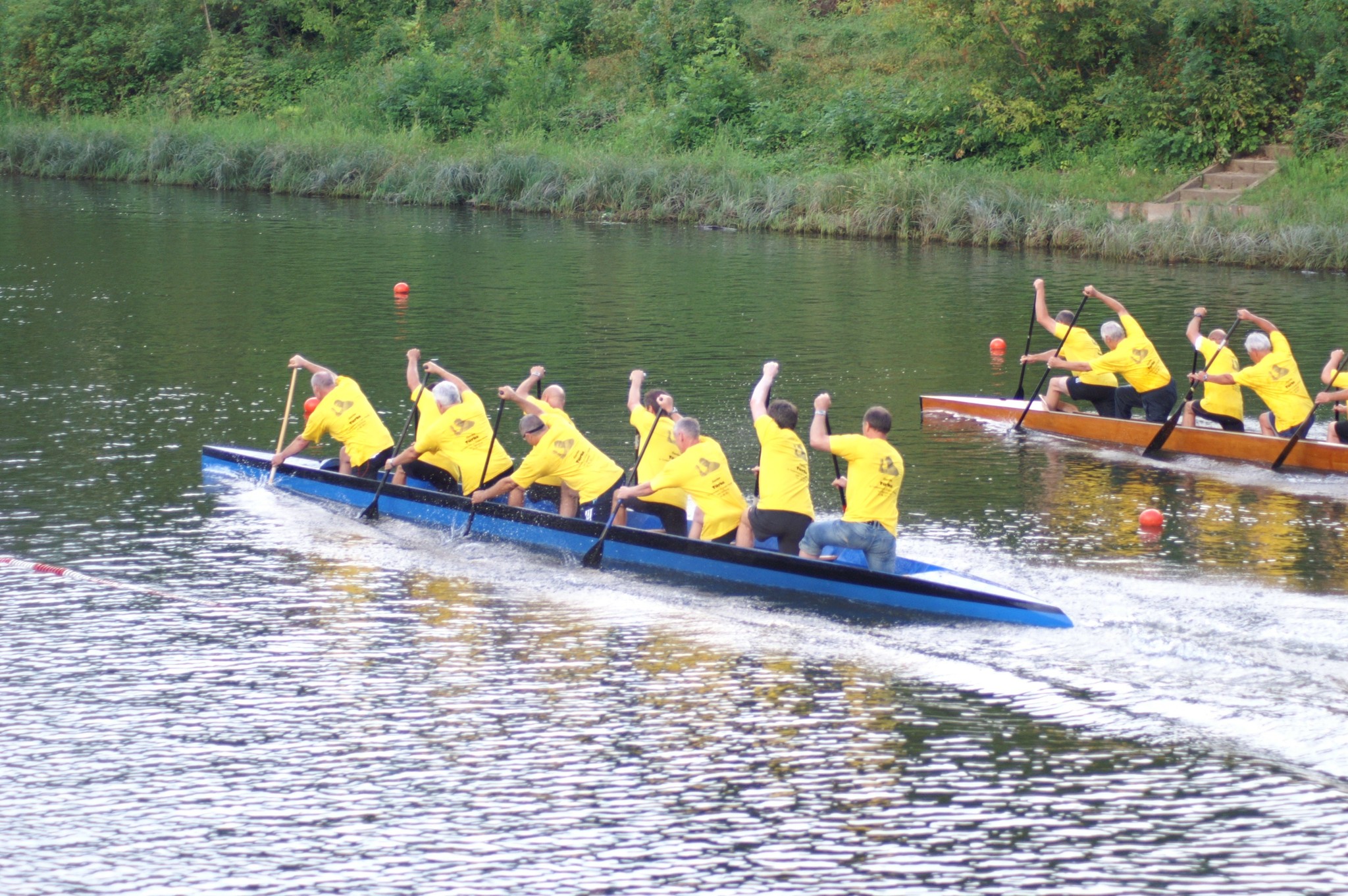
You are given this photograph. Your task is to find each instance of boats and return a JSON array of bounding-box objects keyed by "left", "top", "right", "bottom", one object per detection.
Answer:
[
  {"left": 918, "top": 394, "right": 1348, "bottom": 476},
  {"left": 199, "top": 443, "right": 1074, "bottom": 632}
]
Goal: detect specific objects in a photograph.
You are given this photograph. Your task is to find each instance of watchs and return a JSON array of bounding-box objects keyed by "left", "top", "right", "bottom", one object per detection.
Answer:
[
  {"left": 815, "top": 410, "right": 826, "bottom": 415},
  {"left": 1193, "top": 313, "right": 1203, "bottom": 319},
  {"left": 668, "top": 407, "right": 678, "bottom": 417},
  {"left": 1203, "top": 374, "right": 1208, "bottom": 381}
]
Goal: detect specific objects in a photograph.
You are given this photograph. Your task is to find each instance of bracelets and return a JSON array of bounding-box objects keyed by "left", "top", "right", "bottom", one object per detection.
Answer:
[{"left": 532, "top": 372, "right": 541, "bottom": 378}]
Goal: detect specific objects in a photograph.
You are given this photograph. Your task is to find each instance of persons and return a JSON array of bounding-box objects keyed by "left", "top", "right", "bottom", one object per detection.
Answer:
[
  {"left": 736, "top": 361, "right": 815, "bottom": 556},
  {"left": 1045, "top": 285, "right": 1177, "bottom": 423},
  {"left": 1181, "top": 307, "right": 1244, "bottom": 432},
  {"left": 1020, "top": 279, "right": 1118, "bottom": 418},
  {"left": 272, "top": 355, "right": 395, "bottom": 480},
  {"left": 508, "top": 366, "right": 580, "bottom": 518},
  {"left": 799, "top": 393, "right": 904, "bottom": 574},
  {"left": 618, "top": 394, "right": 747, "bottom": 544},
  {"left": 391, "top": 348, "right": 458, "bottom": 495},
  {"left": 384, "top": 361, "right": 514, "bottom": 497},
  {"left": 1187, "top": 309, "right": 1315, "bottom": 439},
  {"left": 611, "top": 370, "right": 688, "bottom": 538},
  {"left": 471, "top": 386, "right": 626, "bottom": 523},
  {"left": 1314, "top": 349, "right": 1348, "bottom": 443}
]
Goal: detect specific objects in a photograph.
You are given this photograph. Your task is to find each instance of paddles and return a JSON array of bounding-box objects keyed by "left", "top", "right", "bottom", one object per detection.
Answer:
[
  {"left": 265, "top": 365, "right": 299, "bottom": 488},
  {"left": 1006, "top": 291, "right": 1092, "bottom": 437},
  {"left": 581, "top": 395, "right": 670, "bottom": 567},
  {"left": 1012, "top": 291, "right": 1044, "bottom": 403},
  {"left": 461, "top": 389, "right": 508, "bottom": 538},
  {"left": 752, "top": 367, "right": 777, "bottom": 507},
  {"left": 356, "top": 362, "right": 438, "bottom": 521},
  {"left": 1185, "top": 307, "right": 1208, "bottom": 404},
  {"left": 823, "top": 409, "right": 846, "bottom": 514},
  {"left": 1270, "top": 355, "right": 1348, "bottom": 472},
  {"left": 1142, "top": 314, "right": 1244, "bottom": 458}
]
